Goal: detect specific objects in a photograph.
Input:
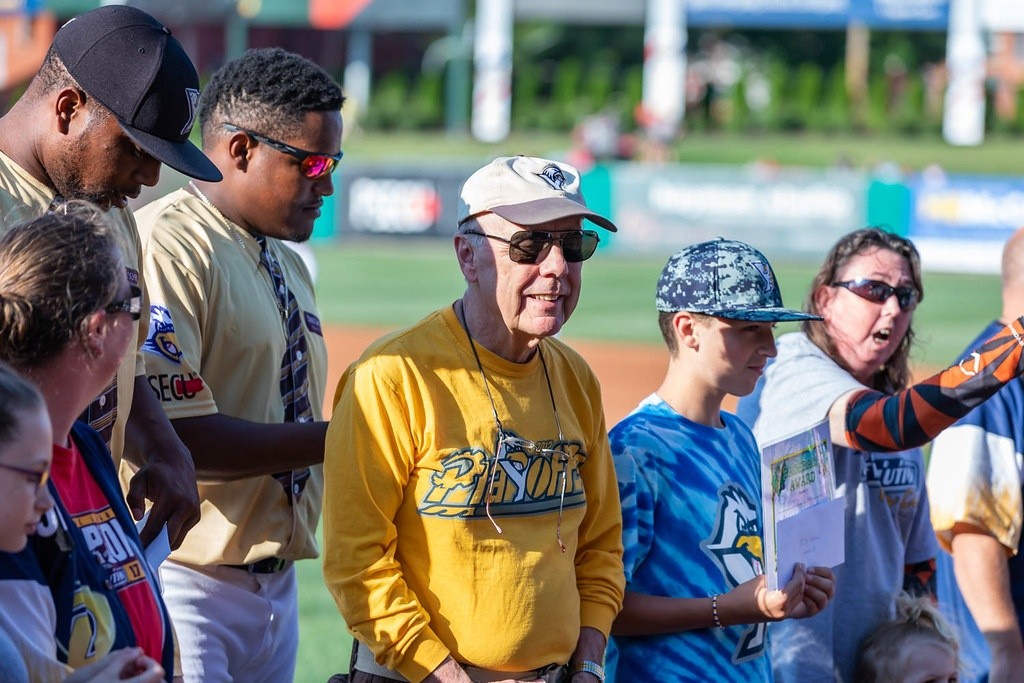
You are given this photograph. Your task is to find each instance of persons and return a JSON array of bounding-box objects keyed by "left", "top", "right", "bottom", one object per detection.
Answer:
[
  {"left": 736, "top": 228, "right": 1024, "bottom": 683},
  {"left": 0, "top": 4, "right": 223, "bottom": 683},
  {"left": 927, "top": 370, "right": 1024, "bottom": 683},
  {"left": 855, "top": 597, "right": 959, "bottom": 683},
  {"left": 324, "top": 157, "right": 625, "bottom": 683},
  {"left": 603, "top": 237, "right": 835, "bottom": 683},
  {"left": 118, "top": 48, "right": 331, "bottom": 683}
]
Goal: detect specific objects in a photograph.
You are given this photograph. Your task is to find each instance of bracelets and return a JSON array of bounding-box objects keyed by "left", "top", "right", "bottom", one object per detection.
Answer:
[
  {"left": 569, "top": 661, "right": 604, "bottom": 681},
  {"left": 713, "top": 593, "right": 726, "bottom": 631}
]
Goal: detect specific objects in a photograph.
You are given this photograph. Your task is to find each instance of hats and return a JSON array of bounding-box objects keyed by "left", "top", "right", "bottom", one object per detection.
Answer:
[
  {"left": 655, "top": 237, "right": 825, "bottom": 322},
  {"left": 53, "top": 5, "right": 223, "bottom": 183},
  {"left": 458, "top": 154, "right": 618, "bottom": 233}
]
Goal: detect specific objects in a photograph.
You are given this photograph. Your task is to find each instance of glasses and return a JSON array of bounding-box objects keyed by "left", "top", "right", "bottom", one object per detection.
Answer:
[
  {"left": 828, "top": 279, "right": 920, "bottom": 313},
  {"left": 220, "top": 122, "right": 343, "bottom": 180},
  {"left": 462, "top": 229, "right": 601, "bottom": 264},
  {"left": 485, "top": 434, "right": 574, "bottom": 553},
  {"left": 61, "top": 285, "right": 142, "bottom": 342},
  {"left": 0, "top": 463, "right": 49, "bottom": 496}
]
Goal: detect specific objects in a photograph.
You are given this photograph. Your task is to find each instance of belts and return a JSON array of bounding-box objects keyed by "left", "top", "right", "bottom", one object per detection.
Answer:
[
  {"left": 354, "top": 638, "right": 570, "bottom": 683},
  {"left": 221, "top": 556, "right": 286, "bottom": 574}
]
